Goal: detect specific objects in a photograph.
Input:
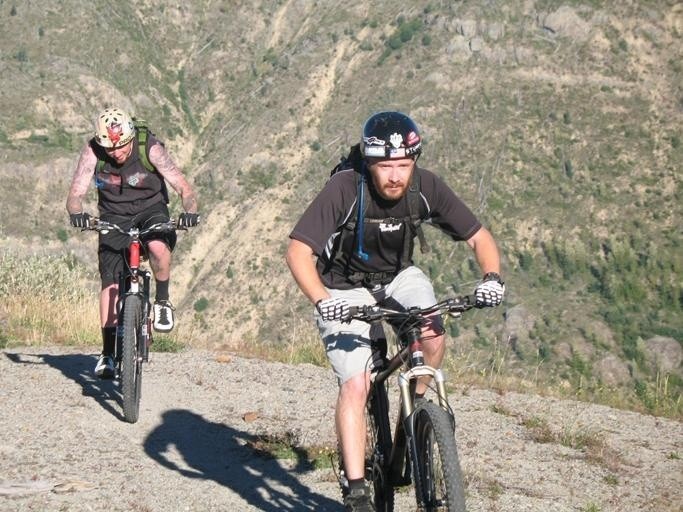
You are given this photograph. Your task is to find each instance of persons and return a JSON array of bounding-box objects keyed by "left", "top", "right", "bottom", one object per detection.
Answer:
[
  {"left": 285, "top": 111, "right": 505, "bottom": 512},
  {"left": 66, "top": 106, "right": 198, "bottom": 376}
]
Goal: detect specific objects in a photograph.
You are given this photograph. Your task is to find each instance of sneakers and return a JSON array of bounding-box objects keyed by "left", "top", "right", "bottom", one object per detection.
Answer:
[
  {"left": 152, "top": 299, "right": 174, "bottom": 333},
  {"left": 344, "top": 486, "right": 376, "bottom": 512},
  {"left": 94, "top": 353, "right": 115, "bottom": 377}
]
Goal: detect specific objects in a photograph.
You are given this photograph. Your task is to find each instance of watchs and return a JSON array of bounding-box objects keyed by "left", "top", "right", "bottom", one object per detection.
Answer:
[{"left": 483, "top": 272, "right": 501, "bottom": 283}]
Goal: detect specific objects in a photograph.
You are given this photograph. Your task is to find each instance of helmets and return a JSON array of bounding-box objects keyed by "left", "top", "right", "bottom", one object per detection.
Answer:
[
  {"left": 359, "top": 110, "right": 422, "bottom": 158},
  {"left": 95, "top": 108, "right": 135, "bottom": 147}
]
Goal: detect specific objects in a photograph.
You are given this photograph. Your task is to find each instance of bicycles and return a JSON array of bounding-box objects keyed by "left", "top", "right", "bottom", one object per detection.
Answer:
[
  {"left": 330, "top": 295, "right": 488, "bottom": 510},
  {"left": 76, "top": 213, "right": 200, "bottom": 424}
]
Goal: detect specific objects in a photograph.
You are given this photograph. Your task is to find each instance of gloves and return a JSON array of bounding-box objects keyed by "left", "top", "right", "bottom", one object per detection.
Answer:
[
  {"left": 474, "top": 272, "right": 505, "bottom": 309},
  {"left": 317, "top": 298, "right": 348, "bottom": 320},
  {"left": 70, "top": 212, "right": 89, "bottom": 228},
  {"left": 177, "top": 213, "right": 199, "bottom": 230}
]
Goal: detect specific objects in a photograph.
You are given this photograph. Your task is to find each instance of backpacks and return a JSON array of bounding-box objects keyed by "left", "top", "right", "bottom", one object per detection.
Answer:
[
  {"left": 95, "top": 116, "right": 157, "bottom": 174},
  {"left": 329, "top": 141, "right": 424, "bottom": 286}
]
